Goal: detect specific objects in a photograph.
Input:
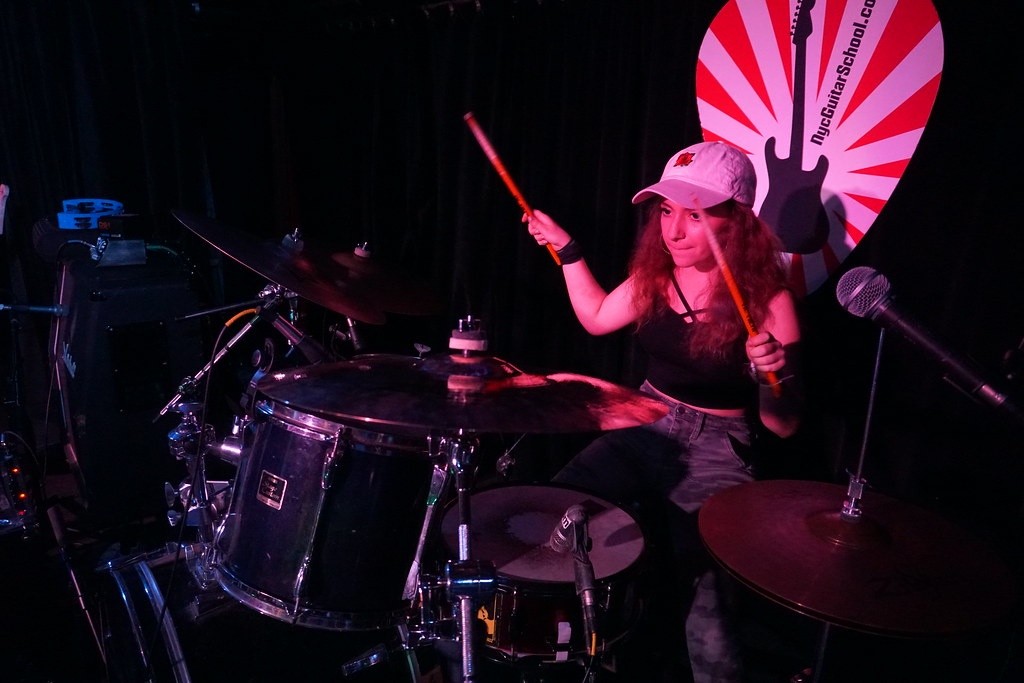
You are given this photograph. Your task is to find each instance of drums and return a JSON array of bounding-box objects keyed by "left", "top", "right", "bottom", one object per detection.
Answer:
[
  {"left": 212, "top": 394, "right": 433, "bottom": 630},
  {"left": 95, "top": 546, "right": 177, "bottom": 683},
  {"left": 433, "top": 482, "right": 650, "bottom": 664},
  {"left": 138, "top": 544, "right": 271, "bottom": 683}
]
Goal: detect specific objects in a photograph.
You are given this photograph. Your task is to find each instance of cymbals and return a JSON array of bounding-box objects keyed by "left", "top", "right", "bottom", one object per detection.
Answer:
[
  {"left": 171, "top": 207, "right": 387, "bottom": 326},
  {"left": 698, "top": 483, "right": 1011, "bottom": 641},
  {"left": 330, "top": 247, "right": 440, "bottom": 316},
  {"left": 256, "top": 355, "right": 671, "bottom": 432}
]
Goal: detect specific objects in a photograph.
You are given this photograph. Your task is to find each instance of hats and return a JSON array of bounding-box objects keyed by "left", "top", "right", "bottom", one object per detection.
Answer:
[{"left": 632, "top": 141, "right": 757, "bottom": 210}]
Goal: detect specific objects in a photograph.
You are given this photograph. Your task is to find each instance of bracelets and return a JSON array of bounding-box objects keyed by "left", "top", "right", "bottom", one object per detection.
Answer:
[{"left": 557, "top": 238, "right": 582, "bottom": 264}]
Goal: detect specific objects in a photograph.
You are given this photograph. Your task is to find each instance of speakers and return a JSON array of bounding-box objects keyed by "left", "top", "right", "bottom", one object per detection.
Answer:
[{"left": 49, "top": 257, "right": 203, "bottom": 516}]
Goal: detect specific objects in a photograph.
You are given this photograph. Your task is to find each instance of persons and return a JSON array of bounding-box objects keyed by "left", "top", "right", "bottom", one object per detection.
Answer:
[{"left": 522, "top": 141, "right": 803, "bottom": 683}]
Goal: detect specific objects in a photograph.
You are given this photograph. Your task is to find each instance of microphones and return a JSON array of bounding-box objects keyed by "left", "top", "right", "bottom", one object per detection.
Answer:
[
  {"left": 256, "top": 305, "right": 335, "bottom": 367},
  {"left": 835, "top": 266, "right": 1007, "bottom": 411},
  {"left": 549, "top": 505, "right": 587, "bottom": 554},
  {"left": 0, "top": 304, "right": 63, "bottom": 317},
  {"left": 347, "top": 315, "right": 362, "bottom": 352}
]
